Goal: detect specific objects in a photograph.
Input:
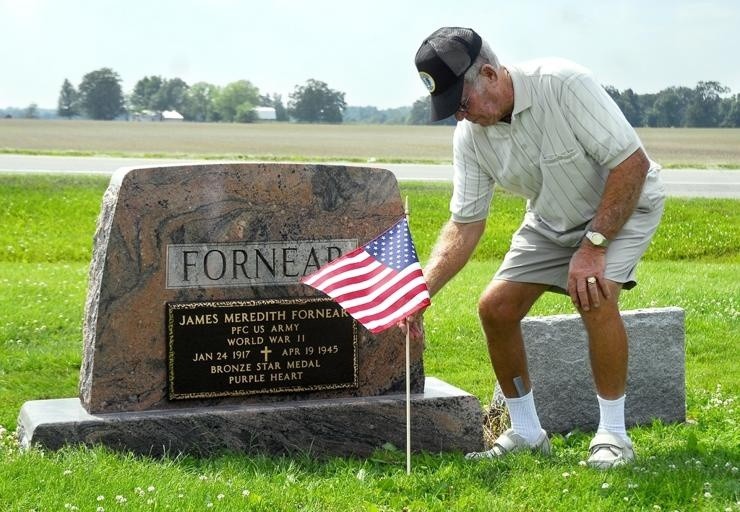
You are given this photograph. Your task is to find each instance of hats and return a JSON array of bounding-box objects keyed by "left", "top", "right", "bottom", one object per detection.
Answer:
[{"left": 415, "top": 26, "right": 483, "bottom": 121}]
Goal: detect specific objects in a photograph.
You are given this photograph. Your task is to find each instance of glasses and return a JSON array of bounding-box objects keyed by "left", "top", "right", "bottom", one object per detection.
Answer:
[{"left": 458, "top": 86, "right": 473, "bottom": 111}]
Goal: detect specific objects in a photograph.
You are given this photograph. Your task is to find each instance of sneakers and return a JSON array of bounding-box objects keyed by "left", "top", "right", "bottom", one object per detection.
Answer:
[
  {"left": 464, "top": 428, "right": 551, "bottom": 462},
  {"left": 586, "top": 432, "right": 633, "bottom": 468}
]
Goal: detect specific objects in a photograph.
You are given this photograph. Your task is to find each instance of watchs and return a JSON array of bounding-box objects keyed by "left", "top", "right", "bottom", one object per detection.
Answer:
[{"left": 586, "top": 231, "right": 609, "bottom": 249}]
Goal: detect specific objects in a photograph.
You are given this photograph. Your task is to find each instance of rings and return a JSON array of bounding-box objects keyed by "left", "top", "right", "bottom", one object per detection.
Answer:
[{"left": 588, "top": 277, "right": 597, "bottom": 283}]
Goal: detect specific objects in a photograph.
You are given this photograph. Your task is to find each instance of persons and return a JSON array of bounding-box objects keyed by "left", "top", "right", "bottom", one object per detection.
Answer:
[
  {"left": 397, "top": 27, "right": 665, "bottom": 470},
  {"left": 301, "top": 217, "right": 431, "bottom": 334}
]
